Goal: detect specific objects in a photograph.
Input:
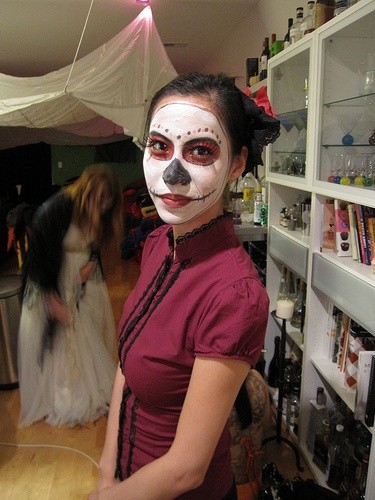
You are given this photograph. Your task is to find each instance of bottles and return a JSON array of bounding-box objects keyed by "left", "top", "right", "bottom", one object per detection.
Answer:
[
  {"left": 278, "top": 267, "right": 307, "bottom": 329},
  {"left": 258, "top": 0, "right": 360, "bottom": 82},
  {"left": 267, "top": 335, "right": 373, "bottom": 500},
  {"left": 232, "top": 171, "right": 264, "bottom": 226}
]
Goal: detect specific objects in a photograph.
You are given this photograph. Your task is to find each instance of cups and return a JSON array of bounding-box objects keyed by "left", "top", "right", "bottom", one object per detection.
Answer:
[
  {"left": 362, "top": 70, "right": 375, "bottom": 94},
  {"left": 329, "top": 152, "right": 375, "bottom": 184}
]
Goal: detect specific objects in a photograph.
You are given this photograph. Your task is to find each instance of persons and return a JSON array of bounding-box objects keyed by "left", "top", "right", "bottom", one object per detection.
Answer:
[
  {"left": 19, "top": 163, "right": 124, "bottom": 428},
  {"left": 89, "top": 69, "right": 281, "bottom": 500}
]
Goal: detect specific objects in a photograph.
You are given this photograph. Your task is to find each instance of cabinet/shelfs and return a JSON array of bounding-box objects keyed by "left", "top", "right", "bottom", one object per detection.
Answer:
[{"left": 233, "top": 0, "right": 375, "bottom": 500}]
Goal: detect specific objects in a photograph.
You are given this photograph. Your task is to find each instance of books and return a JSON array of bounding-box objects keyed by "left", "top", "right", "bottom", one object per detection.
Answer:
[{"left": 322, "top": 194, "right": 375, "bottom": 428}]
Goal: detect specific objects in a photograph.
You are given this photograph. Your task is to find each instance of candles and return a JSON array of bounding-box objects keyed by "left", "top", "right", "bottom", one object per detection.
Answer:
[{"left": 276, "top": 298, "right": 294, "bottom": 319}]
